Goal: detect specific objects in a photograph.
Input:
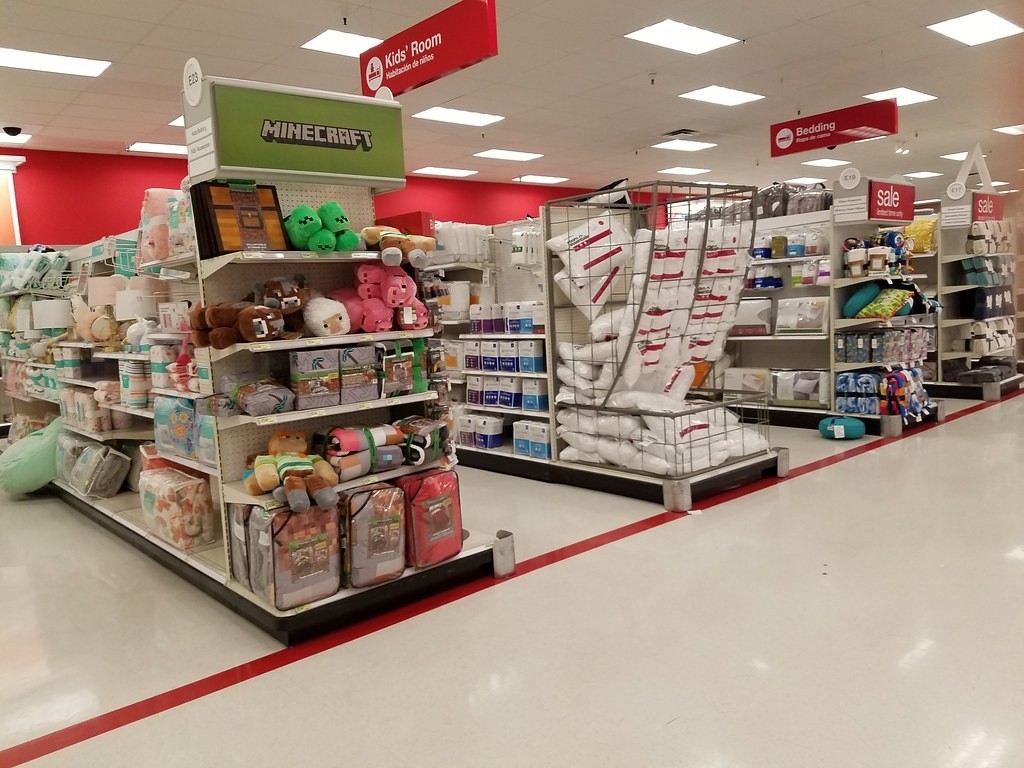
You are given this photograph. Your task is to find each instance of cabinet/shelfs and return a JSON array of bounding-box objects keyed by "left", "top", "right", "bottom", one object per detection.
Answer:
[
  {"left": 424, "top": 262, "right": 553, "bottom": 484},
  {"left": 0, "top": 228, "right": 228, "bottom": 615},
  {"left": 547, "top": 181, "right": 774, "bottom": 479},
  {"left": 199, "top": 250, "right": 494, "bottom": 647},
  {"left": 723, "top": 209, "right": 1024, "bottom": 439}
]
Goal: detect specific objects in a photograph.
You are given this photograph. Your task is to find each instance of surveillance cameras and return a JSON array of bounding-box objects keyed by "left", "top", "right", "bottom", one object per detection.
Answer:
[
  {"left": 3, "top": 127, "right": 21, "bottom": 136},
  {"left": 827, "top": 146, "right": 837, "bottom": 150}
]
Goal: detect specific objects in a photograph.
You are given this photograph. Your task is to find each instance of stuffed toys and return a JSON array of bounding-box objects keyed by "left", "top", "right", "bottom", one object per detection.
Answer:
[
  {"left": 190, "top": 275, "right": 350, "bottom": 349},
  {"left": 362, "top": 225, "right": 436, "bottom": 269},
  {"left": 243, "top": 430, "right": 339, "bottom": 512},
  {"left": 283, "top": 200, "right": 359, "bottom": 252},
  {"left": 332, "top": 260, "right": 428, "bottom": 333}
]
